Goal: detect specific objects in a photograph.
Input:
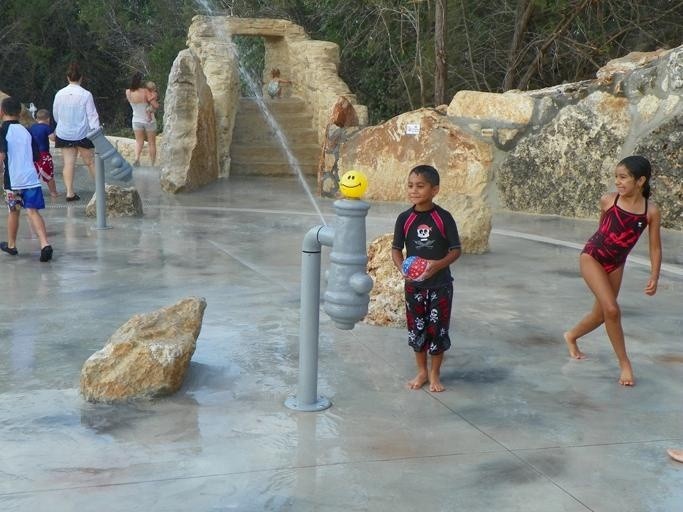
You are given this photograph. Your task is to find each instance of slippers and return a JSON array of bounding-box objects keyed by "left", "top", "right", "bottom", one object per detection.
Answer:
[{"left": 66, "top": 194, "right": 80, "bottom": 201}]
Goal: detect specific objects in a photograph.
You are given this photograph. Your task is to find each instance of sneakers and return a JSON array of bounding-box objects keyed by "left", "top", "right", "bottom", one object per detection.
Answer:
[
  {"left": 0, "top": 242, "right": 17, "bottom": 255},
  {"left": 40, "top": 245, "right": 53, "bottom": 262}
]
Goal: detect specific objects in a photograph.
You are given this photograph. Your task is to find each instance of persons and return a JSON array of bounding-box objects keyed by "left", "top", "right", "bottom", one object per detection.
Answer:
[
  {"left": 262, "top": 68, "right": 292, "bottom": 99},
  {"left": 125, "top": 72, "right": 157, "bottom": 168},
  {"left": 0, "top": 97, "right": 53, "bottom": 262},
  {"left": 391, "top": 164, "right": 462, "bottom": 392},
  {"left": 30, "top": 108, "right": 58, "bottom": 197},
  {"left": 146, "top": 81, "right": 159, "bottom": 122},
  {"left": 563, "top": 156, "right": 661, "bottom": 387},
  {"left": 53, "top": 63, "right": 100, "bottom": 201}
]
